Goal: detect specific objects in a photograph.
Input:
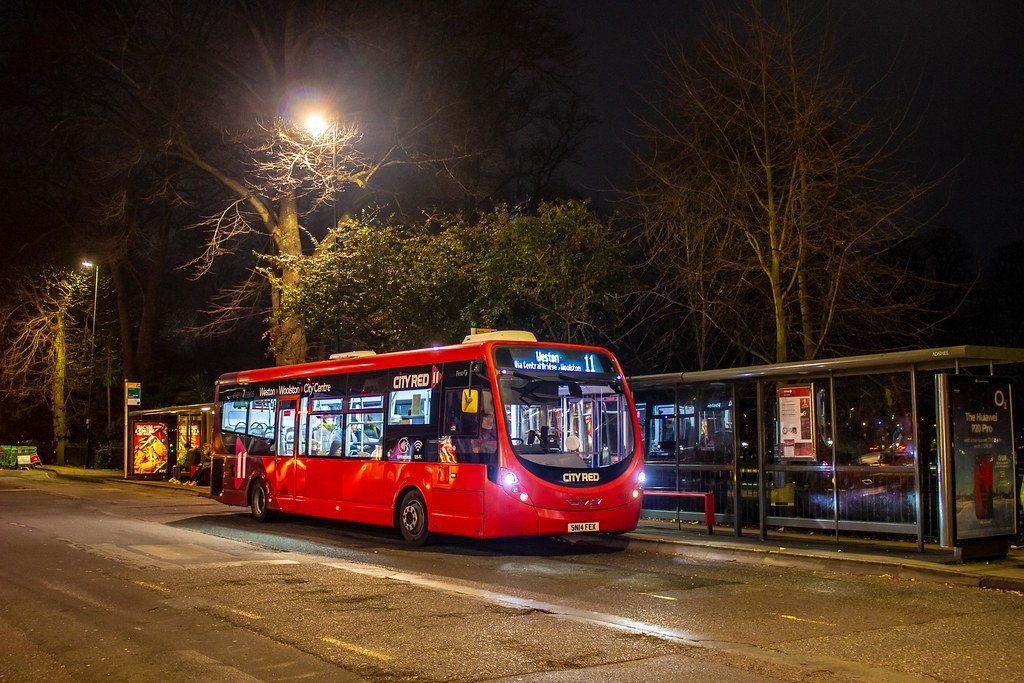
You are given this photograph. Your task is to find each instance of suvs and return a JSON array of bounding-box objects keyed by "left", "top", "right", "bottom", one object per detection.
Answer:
[{"left": 630, "top": 378, "right": 904, "bottom": 512}]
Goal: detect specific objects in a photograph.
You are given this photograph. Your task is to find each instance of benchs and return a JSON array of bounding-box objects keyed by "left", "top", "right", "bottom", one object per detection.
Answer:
[
  {"left": 641, "top": 486, "right": 716, "bottom": 536},
  {"left": 172, "top": 465, "right": 210, "bottom": 483}
]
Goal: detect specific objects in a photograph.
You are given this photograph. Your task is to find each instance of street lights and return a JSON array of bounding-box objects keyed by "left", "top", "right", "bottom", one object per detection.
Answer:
[{"left": 80, "top": 259, "right": 98, "bottom": 468}]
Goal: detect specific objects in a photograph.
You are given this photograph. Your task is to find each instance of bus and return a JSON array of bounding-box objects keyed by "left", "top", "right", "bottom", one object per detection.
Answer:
[{"left": 208, "top": 327, "right": 644, "bottom": 546}]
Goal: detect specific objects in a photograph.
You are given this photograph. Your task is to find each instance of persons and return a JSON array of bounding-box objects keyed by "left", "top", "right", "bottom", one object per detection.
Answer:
[
  {"left": 301, "top": 415, "right": 383, "bottom": 458},
  {"left": 168, "top": 440, "right": 198, "bottom": 485},
  {"left": 185, "top": 442, "right": 214, "bottom": 485},
  {"left": 471, "top": 413, "right": 498, "bottom": 454}
]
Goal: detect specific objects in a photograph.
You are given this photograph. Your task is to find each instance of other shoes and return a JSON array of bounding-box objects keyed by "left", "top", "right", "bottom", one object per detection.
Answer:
[
  {"left": 188, "top": 481, "right": 198, "bottom": 486},
  {"left": 183, "top": 481, "right": 191, "bottom": 486},
  {"left": 173, "top": 480, "right": 181, "bottom": 484},
  {"left": 169, "top": 477, "right": 176, "bottom": 482}
]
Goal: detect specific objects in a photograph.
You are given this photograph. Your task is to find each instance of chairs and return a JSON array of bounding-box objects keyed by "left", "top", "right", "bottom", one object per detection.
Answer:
[
  {"left": 641, "top": 426, "right": 732, "bottom": 463},
  {"left": 226, "top": 422, "right": 472, "bottom": 459}
]
